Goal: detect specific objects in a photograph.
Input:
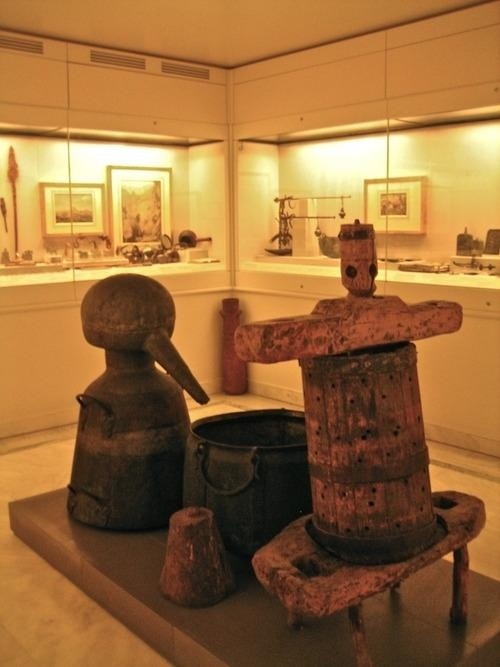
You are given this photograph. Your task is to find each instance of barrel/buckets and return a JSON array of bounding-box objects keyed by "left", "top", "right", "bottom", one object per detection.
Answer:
[{"left": 180, "top": 407, "right": 312, "bottom": 578}]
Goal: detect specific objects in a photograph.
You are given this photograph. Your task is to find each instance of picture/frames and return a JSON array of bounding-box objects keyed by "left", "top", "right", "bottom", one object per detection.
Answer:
[
  {"left": 39, "top": 182, "right": 107, "bottom": 238},
  {"left": 107, "top": 165, "right": 173, "bottom": 256},
  {"left": 363, "top": 177, "right": 427, "bottom": 235}
]
